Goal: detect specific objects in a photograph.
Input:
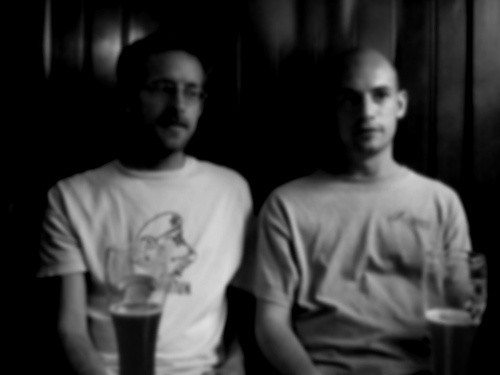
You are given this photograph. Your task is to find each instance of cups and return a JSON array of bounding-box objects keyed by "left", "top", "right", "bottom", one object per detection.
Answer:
[
  {"left": 103, "top": 240, "right": 167, "bottom": 374},
  {"left": 418, "top": 246, "right": 488, "bottom": 374}
]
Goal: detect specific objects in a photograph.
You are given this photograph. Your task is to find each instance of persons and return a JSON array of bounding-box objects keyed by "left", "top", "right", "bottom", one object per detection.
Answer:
[
  {"left": 230, "top": 40, "right": 477, "bottom": 375},
  {"left": 42, "top": 31, "right": 258, "bottom": 375}
]
